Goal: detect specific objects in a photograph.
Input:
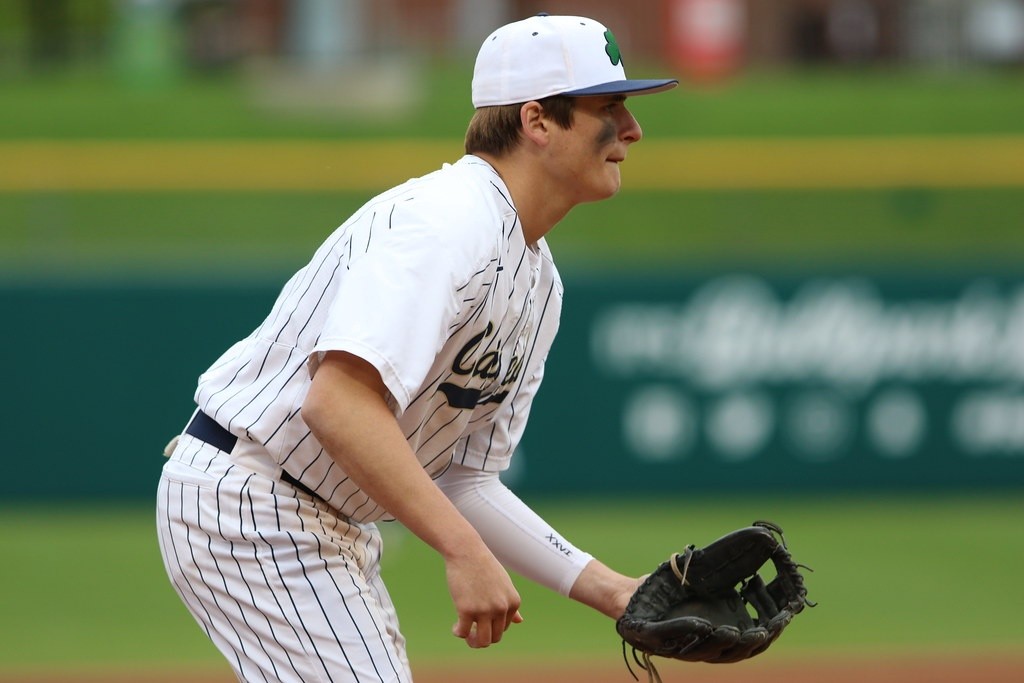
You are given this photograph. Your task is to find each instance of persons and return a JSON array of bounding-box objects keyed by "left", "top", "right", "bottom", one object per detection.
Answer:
[{"left": 154, "top": 15, "right": 808, "bottom": 683}]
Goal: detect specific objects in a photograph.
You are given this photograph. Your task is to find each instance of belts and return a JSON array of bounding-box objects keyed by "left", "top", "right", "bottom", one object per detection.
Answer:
[{"left": 186, "top": 408, "right": 326, "bottom": 504}]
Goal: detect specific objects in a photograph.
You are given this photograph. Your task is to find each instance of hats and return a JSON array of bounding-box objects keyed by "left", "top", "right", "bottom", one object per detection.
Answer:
[{"left": 472, "top": 13, "right": 679, "bottom": 109}]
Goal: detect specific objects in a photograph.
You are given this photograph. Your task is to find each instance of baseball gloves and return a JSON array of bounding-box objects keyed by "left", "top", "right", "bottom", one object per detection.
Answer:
[{"left": 616, "top": 521, "right": 809, "bottom": 664}]
